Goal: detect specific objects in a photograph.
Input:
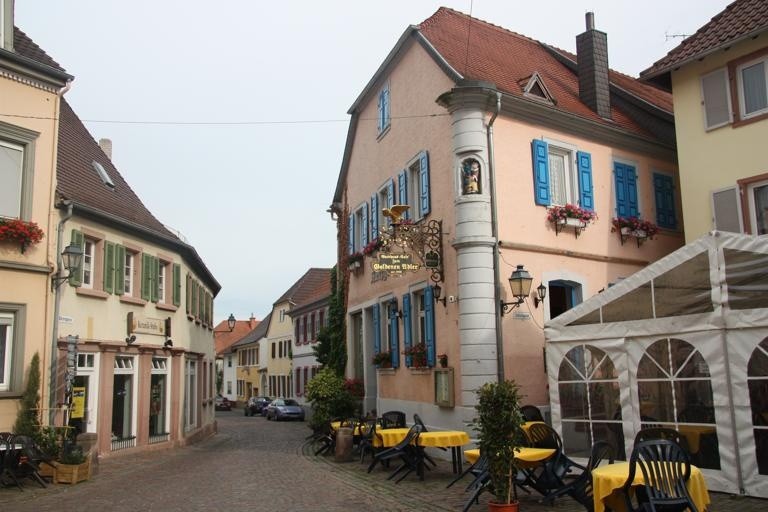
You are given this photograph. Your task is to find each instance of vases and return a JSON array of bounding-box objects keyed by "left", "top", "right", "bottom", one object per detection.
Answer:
[
  {"left": 346, "top": 260, "right": 361, "bottom": 272},
  {"left": 557, "top": 217, "right": 585, "bottom": 228},
  {"left": 620, "top": 225, "right": 646, "bottom": 239}
]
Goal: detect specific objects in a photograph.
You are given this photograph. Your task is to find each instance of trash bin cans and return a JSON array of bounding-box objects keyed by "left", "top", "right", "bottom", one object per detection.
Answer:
[{"left": 335, "top": 427, "right": 354, "bottom": 463}]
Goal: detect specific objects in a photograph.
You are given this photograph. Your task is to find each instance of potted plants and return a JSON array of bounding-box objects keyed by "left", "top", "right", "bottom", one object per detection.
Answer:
[
  {"left": 461, "top": 377, "right": 529, "bottom": 511},
  {"left": 52, "top": 426, "right": 94, "bottom": 485},
  {"left": 12, "top": 348, "right": 57, "bottom": 477}
]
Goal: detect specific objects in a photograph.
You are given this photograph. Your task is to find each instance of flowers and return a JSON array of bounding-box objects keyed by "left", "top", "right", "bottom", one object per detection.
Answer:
[
  {"left": 436, "top": 353, "right": 448, "bottom": 369},
  {"left": 0, "top": 213, "right": 47, "bottom": 256},
  {"left": 370, "top": 347, "right": 393, "bottom": 367},
  {"left": 611, "top": 215, "right": 661, "bottom": 243},
  {"left": 342, "top": 377, "right": 364, "bottom": 401},
  {"left": 398, "top": 336, "right": 431, "bottom": 371},
  {"left": 340, "top": 250, "right": 363, "bottom": 272},
  {"left": 364, "top": 237, "right": 386, "bottom": 260},
  {"left": 545, "top": 197, "right": 601, "bottom": 232}
]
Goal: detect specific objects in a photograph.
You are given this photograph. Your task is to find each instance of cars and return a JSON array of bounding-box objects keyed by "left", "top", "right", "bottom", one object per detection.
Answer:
[
  {"left": 263, "top": 398, "right": 306, "bottom": 421},
  {"left": 215, "top": 398, "right": 231, "bottom": 411},
  {"left": 241, "top": 394, "right": 271, "bottom": 417}
]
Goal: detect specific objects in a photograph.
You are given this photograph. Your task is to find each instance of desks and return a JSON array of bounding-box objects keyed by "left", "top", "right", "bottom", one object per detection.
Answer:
[
  {"left": 410, "top": 431, "right": 467, "bottom": 481},
  {"left": 592, "top": 461, "right": 701, "bottom": 511}
]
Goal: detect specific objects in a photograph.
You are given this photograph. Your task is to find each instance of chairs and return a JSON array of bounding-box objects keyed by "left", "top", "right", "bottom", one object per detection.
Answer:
[
  {"left": 447, "top": 406, "right": 614, "bottom": 511},
  {"left": 613, "top": 440, "right": 697, "bottom": 510},
  {"left": 311, "top": 411, "right": 447, "bottom": 484},
  {"left": 0, "top": 430, "right": 58, "bottom": 493},
  {"left": 634, "top": 428, "right": 691, "bottom": 462}
]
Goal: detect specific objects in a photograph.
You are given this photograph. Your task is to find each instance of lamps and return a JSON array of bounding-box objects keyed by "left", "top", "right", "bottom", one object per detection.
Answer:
[
  {"left": 534, "top": 280, "right": 546, "bottom": 310},
  {"left": 433, "top": 282, "right": 446, "bottom": 309},
  {"left": 51, "top": 238, "right": 83, "bottom": 295},
  {"left": 213, "top": 313, "right": 237, "bottom": 336},
  {"left": 500, "top": 263, "right": 534, "bottom": 316},
  {"left": 390, "top": 295, "right": 402, "bottom": 319}
]
